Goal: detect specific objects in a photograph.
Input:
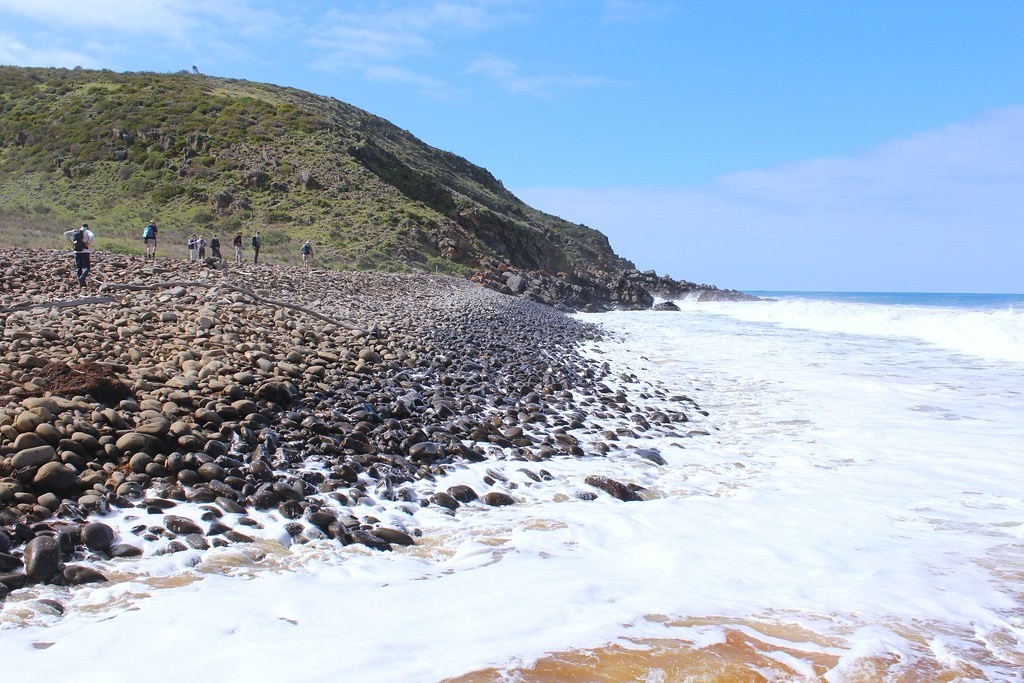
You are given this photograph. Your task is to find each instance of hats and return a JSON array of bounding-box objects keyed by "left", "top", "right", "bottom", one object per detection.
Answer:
[
  {"left": 237, "top": 231, "right": 242, "bottom": 233},
  {"left": 83, "top": 224, "right": 88, "bottom": 227},
  {"left": 150, "top": 220, "right": 156, "bottom": 224},
  {"left": 306, "top": 240, "right": 311, "bottom": 243}
]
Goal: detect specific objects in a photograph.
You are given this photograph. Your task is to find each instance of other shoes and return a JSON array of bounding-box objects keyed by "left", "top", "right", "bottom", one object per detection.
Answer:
[{"left": 78, "top": 278, "right": 87, "bottom": 287}]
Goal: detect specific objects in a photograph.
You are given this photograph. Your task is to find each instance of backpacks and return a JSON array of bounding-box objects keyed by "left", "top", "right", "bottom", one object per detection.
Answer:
[
  {"left": 73, "top": 228, "right": 87, "bottom": 251},
  {"left": 303, "top": 245, "right": 310, "bottom": 255},
  {"left": 199, "top": 239, "right": 204, "bottom": 247},
  {"left": 143, "top": 225, "right": 155, "bottom": 237}
]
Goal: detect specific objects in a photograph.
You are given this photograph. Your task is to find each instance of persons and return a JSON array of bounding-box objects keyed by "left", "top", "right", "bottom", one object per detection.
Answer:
[
  {"left": 142, "top": 219, "right": 157, "bottom": 260},
  {"left": 188, "top": 234, "right": 207, "bottom": 261},
  {"left": 63, "top": 223, "right": 95, "bottom": 288},
  {"left": 232, "top": 230, "right": 244, "bottom": 263},
  {"left": 300, "top": 239, "right": 314, "bottom": 271},
  {"left": 251, "top": 231, "right": 263, "bottom": 264},
  {"left": 210, "top": 234, "right": 222, "bottom": 259}
]
